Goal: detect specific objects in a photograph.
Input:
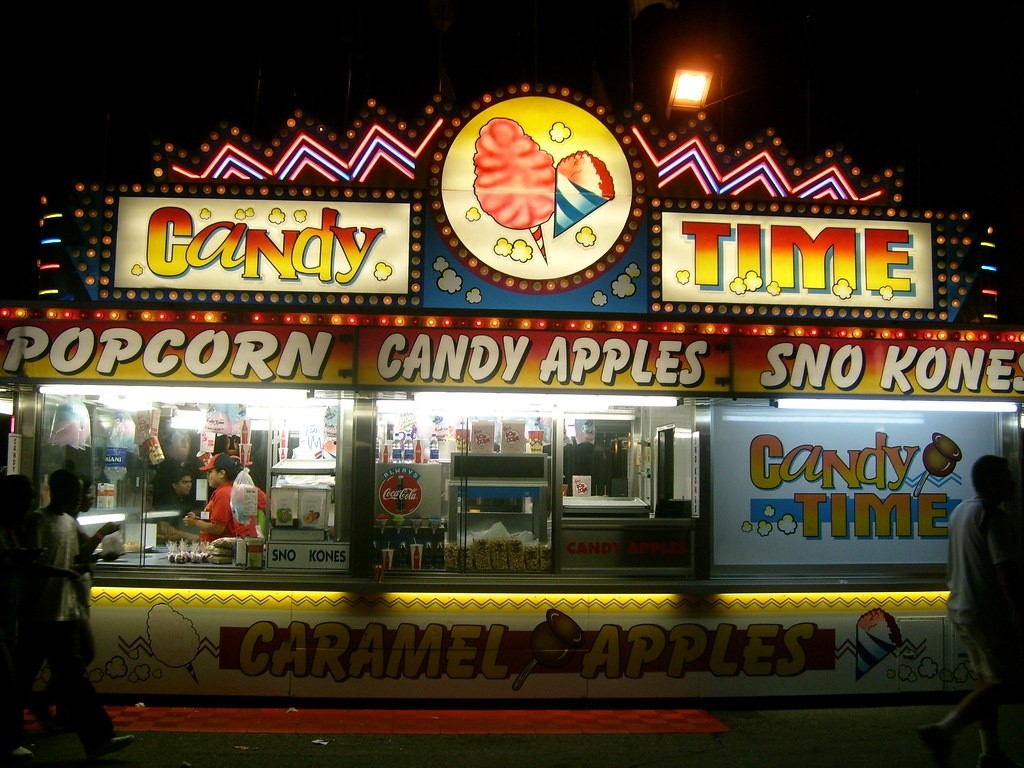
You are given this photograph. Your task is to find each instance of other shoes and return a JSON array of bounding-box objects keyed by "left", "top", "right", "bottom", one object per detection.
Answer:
[
  {"left": 0, "top": 745, "right": 33, "bottom": 756},
  {"left": 87, "top": 734, "right": 136, "bottom": 760},
  {"left": 975, "top": 749, "right": 1024, "bottom": 768},
  {"left": 916, "top": 723, "right": 955, "bottom": 768}
]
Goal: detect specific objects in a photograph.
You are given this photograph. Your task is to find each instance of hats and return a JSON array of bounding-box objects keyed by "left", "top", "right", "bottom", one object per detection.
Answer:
[{"left": 198, "top": 454, "right": 238, "bottom": 476}]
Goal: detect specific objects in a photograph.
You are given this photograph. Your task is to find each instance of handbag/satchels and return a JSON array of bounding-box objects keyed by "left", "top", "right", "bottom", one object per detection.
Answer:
[
  {"left": 46, "top": 619, "right": 94, "bottom": 687},
  {"left": 229, "top": 468, "right": 258, "bottom": 525}
]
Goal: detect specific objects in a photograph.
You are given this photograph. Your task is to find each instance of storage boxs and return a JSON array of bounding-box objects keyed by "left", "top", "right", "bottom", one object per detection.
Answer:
[{"left": 97, "top": 483, "right": 114, "bottom": 509}]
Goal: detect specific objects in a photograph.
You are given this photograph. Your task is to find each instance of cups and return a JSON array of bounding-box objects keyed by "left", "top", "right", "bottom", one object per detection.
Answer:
[
  {"left": 528, "top": 430, "right": 544, "bottom": 454},
  {"left": 380, "top": 444, "right": 391, "bottom": 464},
  {"left": 410, "top": 543, "right": 424, "bottom": 570},
  {"left": 196, "top": 450, "right": 213, "bottom": 466},
  {"left": 456, "top": 429, "right": 471, "bottom": 452},
  {"left": 238, "top": 418, "right": 254, "bottom": 464},
  {"left": 277, "top": 430, "right": 289, "bottom": 461},
  {"left": 381, "top": 549, "right": 394, "bottom": 571},
  {"left": 412, "top": 439, "right": 425, "bottom": 463},
  {"left": 142, "top": 436, "right": 164, "bottom": 465}
]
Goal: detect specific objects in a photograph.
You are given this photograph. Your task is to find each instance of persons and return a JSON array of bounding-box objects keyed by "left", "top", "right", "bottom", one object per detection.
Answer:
[
  {"left": 917, "top": 455, "right": 1024, "bottom": 768},
  {"left": 153, "top": 454, "right": 267, "bottom": 545},
  {"left": 0, "top": 469, "right": 134, "bottom": 768}
]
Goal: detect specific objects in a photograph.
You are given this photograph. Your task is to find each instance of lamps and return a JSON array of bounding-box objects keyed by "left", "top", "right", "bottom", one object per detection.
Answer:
[{"left": 663, "top": 61, "right": 714, "bottom": 119}]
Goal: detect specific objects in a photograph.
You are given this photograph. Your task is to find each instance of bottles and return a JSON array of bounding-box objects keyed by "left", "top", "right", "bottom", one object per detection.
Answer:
[
  {"left": 533, "top": 422, "right": 540, "bottom": 431},
  {"left": 429, "top": 434, "right": 440, "bottom": 464}
]
